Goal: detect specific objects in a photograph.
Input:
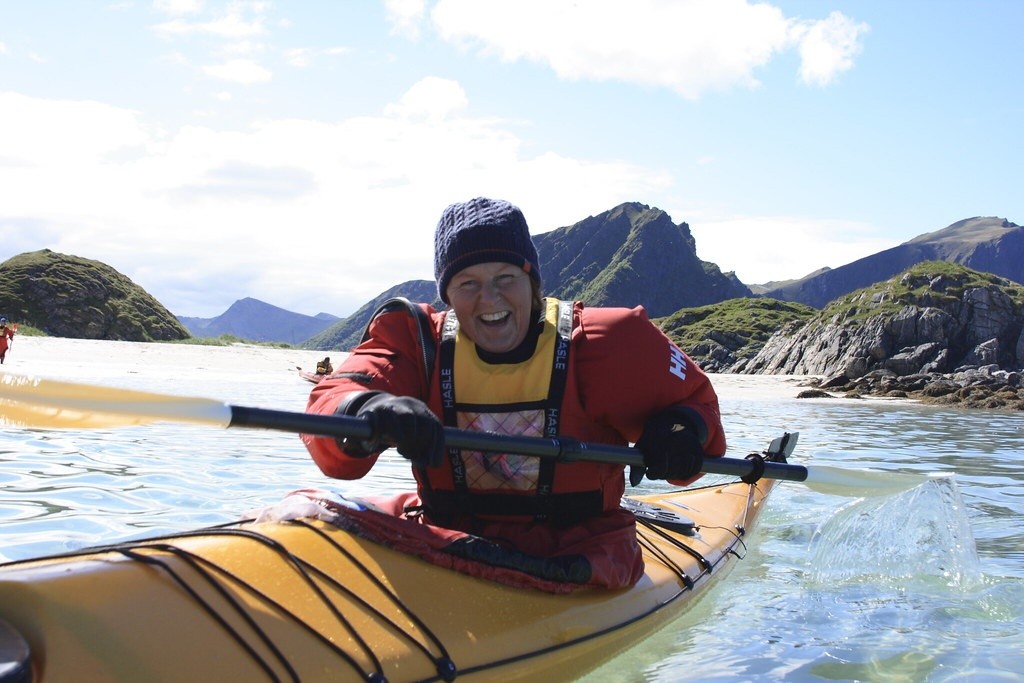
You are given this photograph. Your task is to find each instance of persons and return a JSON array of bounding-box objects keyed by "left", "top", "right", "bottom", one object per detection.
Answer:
[
  {"left": 0, "top": 318, "right": 14, "bottom": 364},
  {"left": 302, "top": 197, "right": 727, "bottom": 591},
  {"left": 316, "top": 357, "right": 333, "bottom": 375}
]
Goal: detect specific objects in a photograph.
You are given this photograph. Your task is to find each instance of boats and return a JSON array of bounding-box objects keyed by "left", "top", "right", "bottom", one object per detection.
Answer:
[
  {"left": 0, "top": 431, "right": 801, "bottom": 683},
  {"left": 295, "top": 365, "right": 326, "bottom": 385}
]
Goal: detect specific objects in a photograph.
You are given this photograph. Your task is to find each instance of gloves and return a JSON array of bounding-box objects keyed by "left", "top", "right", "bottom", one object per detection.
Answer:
[
  {"left": 630, "top": 405, "right": 709, "bottom": 487},
  {"left": 335, "top": 393, "right": 445, "bottom": 468}
]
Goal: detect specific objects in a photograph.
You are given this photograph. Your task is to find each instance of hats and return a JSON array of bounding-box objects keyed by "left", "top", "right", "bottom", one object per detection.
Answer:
[{"left": 434, "top": 197, "right": 540, "bottom": 305}]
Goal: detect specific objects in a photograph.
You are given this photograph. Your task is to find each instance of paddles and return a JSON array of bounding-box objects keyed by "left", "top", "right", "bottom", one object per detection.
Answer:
[
  {"left": 0, "top": 369, "right": 957, "bottom": 499},
  {"left": 8, "top": 324, "right": 18, "bottom": 352}
]
